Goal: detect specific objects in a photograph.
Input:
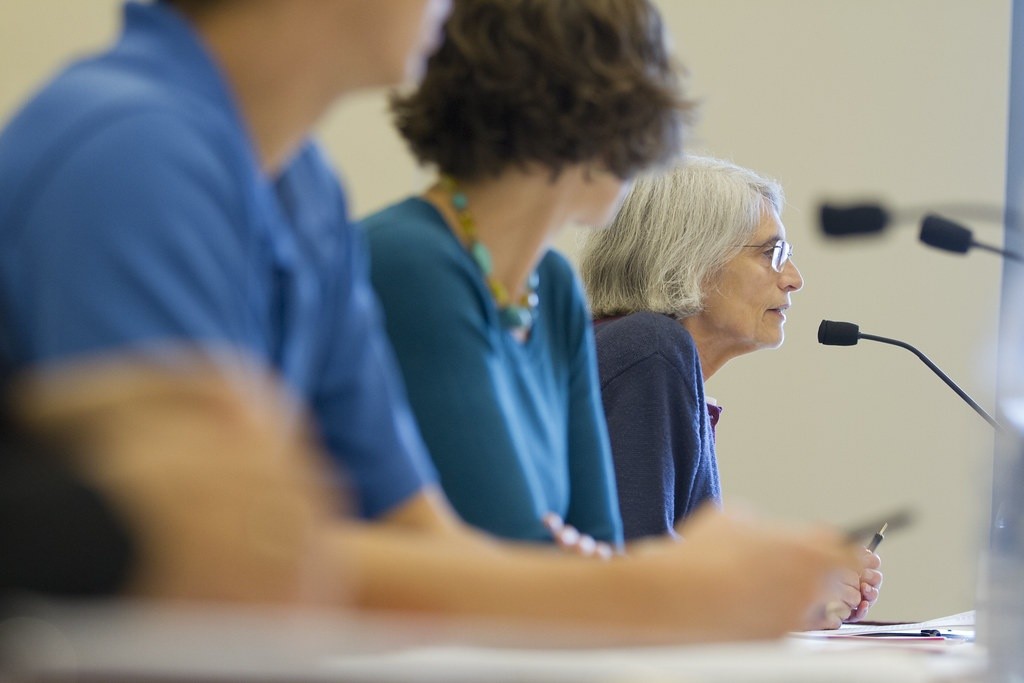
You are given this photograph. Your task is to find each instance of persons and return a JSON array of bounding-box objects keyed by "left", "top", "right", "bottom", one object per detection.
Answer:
[
  {"left": 579, "top": 155, "right": 882, "bottom": 630},
  {"left": 0, "top": 0, "right": 864, "bottom": 651}
]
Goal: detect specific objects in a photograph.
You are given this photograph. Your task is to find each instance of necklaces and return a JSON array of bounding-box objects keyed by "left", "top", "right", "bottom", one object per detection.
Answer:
[{"left": 442, "top": 172, "right": 539, "bottom": 331}]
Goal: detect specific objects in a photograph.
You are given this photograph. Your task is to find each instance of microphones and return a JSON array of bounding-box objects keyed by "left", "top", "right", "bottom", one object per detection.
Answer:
[
  {"left": 818, "top": 320, "right": 1019, "bottom": 451},
  {"left": 820, "top": 201, "right": 890, "bottom": 235},
  {"left": 918, "top": 213, "right": 1024, "bottom": 264}
]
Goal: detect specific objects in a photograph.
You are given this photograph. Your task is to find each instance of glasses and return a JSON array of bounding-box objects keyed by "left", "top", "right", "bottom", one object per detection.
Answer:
[{"left": 743, "top": 239, "right": 794, "bottom": 273}]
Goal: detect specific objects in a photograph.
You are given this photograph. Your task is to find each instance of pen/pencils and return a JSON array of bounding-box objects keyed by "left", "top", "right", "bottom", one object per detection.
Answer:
[{"left": 869, "top": 522, "right": 891, "bottom": 554}]
